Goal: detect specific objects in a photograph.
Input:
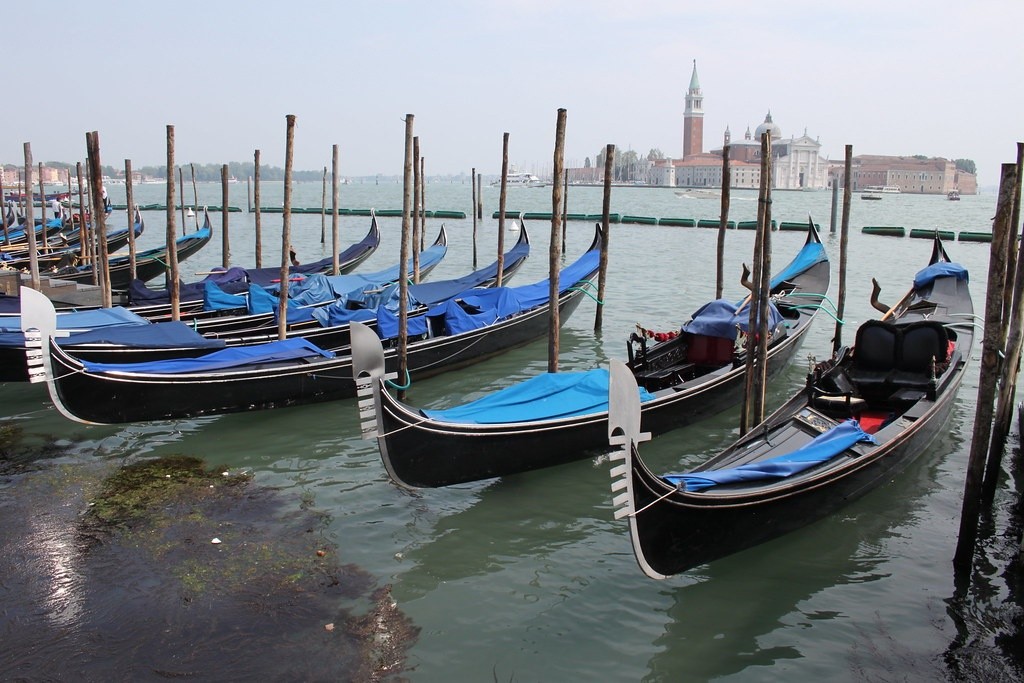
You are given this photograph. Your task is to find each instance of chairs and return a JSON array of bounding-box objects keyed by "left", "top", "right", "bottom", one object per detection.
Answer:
[{"left": 850, "top": 319, "right": 949, "bottom": 398}]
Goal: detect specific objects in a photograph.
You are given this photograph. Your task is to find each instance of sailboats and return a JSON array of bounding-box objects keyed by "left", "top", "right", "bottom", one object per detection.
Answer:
[{"left": 533, "top": 144, "right": 650, "bottom": 185}]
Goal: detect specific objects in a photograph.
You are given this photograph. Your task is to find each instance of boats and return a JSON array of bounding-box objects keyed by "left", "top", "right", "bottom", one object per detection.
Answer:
[
  {"left": 228, "top": 174, "right": 238, "bottom": 183},
  {"left": 2, "top": 177, "right": 168, "bottom": 188},
  {"left": 861, "top": 191, "right": 882, "bottom": 200},
  {"left": 349, "top": 212, "right": 832, "bottom": 493},
  {"left": 1, "top": 185, "right": 606, "bottom": 427},
  {"left": 674, "top": 188, "right": 722, "bottom": 200},
  {"left": 606, "top": 227, "right": 975, "bottom": 580},
  {"left": 947, "top": 187, "right": 961, "bottom": 200},
  {"left": 490, "top": 164, "right": 546, "bottom": 188},
  {"left": 863, "top": 185, "right": 902, "bottom": 194},
  {"left": 340, "top": 176, "right": 352, "bottom": 185}
]
